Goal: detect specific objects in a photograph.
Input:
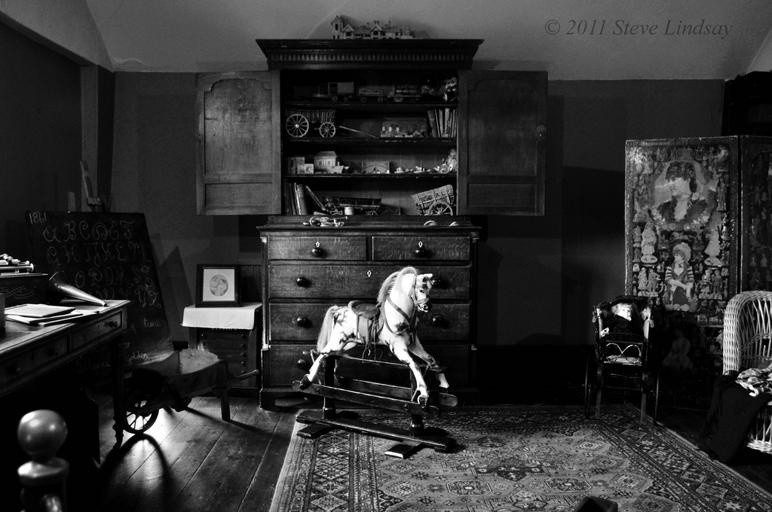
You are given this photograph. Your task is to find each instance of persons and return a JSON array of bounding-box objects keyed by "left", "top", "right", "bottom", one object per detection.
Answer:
[
  {"left": 658, "top": 161, "right": 707, "bottom": 225},
  {"left": 664, "top": 242, "right": 695, "bottom": 306}
]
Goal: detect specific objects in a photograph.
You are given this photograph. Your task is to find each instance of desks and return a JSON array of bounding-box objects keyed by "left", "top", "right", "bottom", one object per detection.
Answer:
[{"left": 0, "top": 272, "right": 132, "bottom": 445}]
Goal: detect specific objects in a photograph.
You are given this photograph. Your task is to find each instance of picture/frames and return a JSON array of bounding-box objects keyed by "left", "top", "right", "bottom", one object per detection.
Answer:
[{"left": 196, "top": 263, "right": 242, "bottom": 309}]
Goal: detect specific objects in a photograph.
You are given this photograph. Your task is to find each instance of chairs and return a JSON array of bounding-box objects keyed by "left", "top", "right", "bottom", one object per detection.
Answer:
[
  {"left": 722, "top": 290, "right": 772, "bottom": 455},
  {"left": 581, "top": 294, "right": 661, "bottom": 426}
]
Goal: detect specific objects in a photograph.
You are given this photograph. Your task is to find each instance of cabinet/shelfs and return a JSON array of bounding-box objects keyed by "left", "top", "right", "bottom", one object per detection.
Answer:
[
  {"left": 196, "top": 38, "right": 548, "bottom": 410},
  {"left": 181, "top": 302, "right": 262, "bottom": 400},
  {"left": 624, "top": 134, "right": 772, "bottom": 414}
]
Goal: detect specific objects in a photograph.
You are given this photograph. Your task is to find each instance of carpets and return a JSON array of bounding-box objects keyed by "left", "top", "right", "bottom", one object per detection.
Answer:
[{"left": 268, "top": 398, "right": 771, "bottom": 512}]
